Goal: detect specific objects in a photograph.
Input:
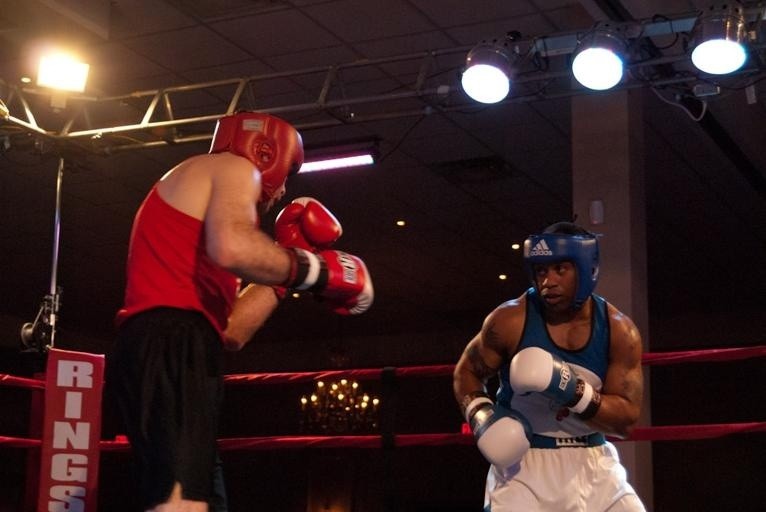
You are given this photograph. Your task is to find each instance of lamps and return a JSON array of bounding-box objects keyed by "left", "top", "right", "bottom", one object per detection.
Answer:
[
  {"left": 297, "top": 133, "right": 384, "bottom": 175},
  {"left": 461, "top": 2, "right": 749, "bottom": 104}
]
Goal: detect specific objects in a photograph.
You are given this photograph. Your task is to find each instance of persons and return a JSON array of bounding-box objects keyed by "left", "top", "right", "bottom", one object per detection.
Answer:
[
  {"left": 452, "top": 218, "right": 657, "bottom": 512},
  {"left": 112, "top": 107, "right": 376, "bottom": 512}
]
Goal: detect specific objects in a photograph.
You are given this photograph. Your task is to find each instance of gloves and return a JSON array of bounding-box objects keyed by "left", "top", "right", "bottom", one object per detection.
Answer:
[
  {"left": 509, "top": 346, "right": 601, "bottom": 420},
  {"left": 273, "top": 197, "right": 343, "bottom": 254},
  {"left": 272, "top": 246, "right": 375, "bottom": 315},
  {"left": 460, "top": 390, "right": 531, "bottom": 469}
]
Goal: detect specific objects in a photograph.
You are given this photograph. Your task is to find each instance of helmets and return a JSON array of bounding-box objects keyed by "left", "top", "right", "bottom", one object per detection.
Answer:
[
  {"left": 522, "top": 222, "right": 600, "bottom": 313},
  {"left": 208, "top": 112, "right": 305, "bottom": 203}
]
